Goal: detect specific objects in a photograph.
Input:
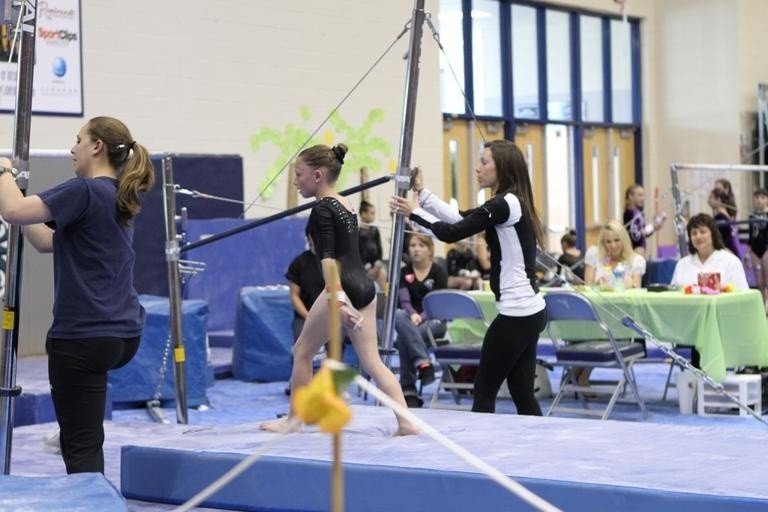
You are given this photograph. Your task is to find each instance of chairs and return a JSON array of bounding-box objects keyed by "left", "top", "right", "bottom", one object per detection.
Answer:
[
  {"left": 420, "top": 288, "right": 490, "bottom": 411},
  {"left": 560, "top": 269, "right": 653, "bottom": 394},
  {"left": 537, "top": 286, "right": 650, "bottom": 421}
]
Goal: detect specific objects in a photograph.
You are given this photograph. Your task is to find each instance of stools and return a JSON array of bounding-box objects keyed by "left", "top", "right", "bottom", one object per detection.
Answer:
[{"left": 661, "top": 344, "right": 700, "bottom": 406}]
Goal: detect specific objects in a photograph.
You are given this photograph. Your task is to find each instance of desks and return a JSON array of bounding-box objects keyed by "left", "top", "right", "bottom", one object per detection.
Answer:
[{"left": 436, "top": 280, "right": 767, "bottom": 374}]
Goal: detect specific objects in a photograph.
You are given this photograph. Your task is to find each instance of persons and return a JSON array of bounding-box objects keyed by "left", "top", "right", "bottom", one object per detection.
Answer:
[
  {"left": 260, "top": 142, "right": 422, "bottom": 438},
  {"left": 389, "top": 140, "right": 547, "bottom": 416},
  {"left": 0, "top": 116, "right": 156, "bottom": 475}
]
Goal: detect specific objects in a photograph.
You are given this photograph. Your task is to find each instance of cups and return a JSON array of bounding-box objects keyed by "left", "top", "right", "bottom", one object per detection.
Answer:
[
  {"left": 698, "top": 272, "right": 721, "bottom": 294},
  {"left": 482, "top": 281, "right": 490, "bottom": 292}
]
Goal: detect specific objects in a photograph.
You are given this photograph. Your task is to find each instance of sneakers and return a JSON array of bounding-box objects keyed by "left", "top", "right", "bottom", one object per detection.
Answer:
[
  {"left": 405, "top": 396, "right": 423, "bottom": 408},
  {"left": 418, "top": 364, "right": 434, "bottom": 385}
]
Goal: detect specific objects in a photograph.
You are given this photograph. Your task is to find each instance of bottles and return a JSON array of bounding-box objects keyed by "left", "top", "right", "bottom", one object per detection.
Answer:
[
  {"left": 614, "top": 261, "right": 626, "bottom": 290},
  {"left": 459, "top": 269, "right": 481, "bottom": 290}
]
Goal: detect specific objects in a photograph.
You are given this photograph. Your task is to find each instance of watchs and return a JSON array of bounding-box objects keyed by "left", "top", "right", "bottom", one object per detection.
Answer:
[{"left": 0, "top": 167, "right": 13, "bottom": 176}]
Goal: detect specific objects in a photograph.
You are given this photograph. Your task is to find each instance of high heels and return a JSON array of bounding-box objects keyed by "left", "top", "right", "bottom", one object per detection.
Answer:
[{"left": 560, "top": 369, "right": 597, "bottom": 399}]
